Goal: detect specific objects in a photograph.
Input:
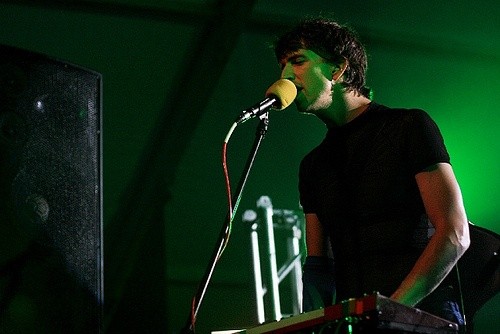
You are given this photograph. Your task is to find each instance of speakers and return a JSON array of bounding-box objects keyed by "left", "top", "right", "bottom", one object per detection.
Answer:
[
  {"left": 0, "top": 42, "right": 105, "bottom": 334},
  {"left": 427, "top": 224, "right": 500, "bottom": 333}
]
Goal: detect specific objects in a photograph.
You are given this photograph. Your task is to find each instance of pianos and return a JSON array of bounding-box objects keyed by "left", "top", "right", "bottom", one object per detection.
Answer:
[{"left": 236, "top": 291, "right": 465, "bottom": 334}]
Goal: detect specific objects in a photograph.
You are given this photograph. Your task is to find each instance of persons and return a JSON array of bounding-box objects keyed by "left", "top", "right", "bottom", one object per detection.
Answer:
[{"left": 274, "top": 16, "right": 471, "bottom": 334}]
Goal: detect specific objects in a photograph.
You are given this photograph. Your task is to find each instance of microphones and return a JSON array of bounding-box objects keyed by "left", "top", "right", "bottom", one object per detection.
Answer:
[{"left": 234, "top": 78, "right": 298, "bottom": 127}]
